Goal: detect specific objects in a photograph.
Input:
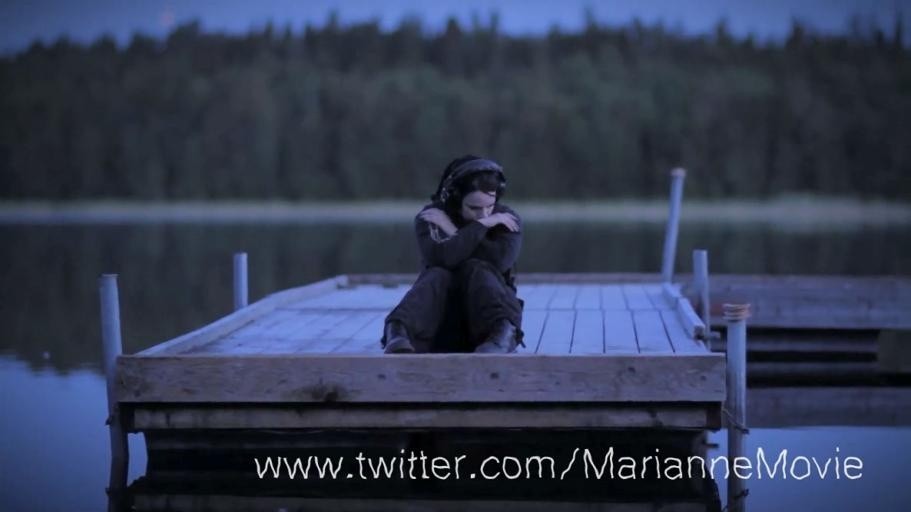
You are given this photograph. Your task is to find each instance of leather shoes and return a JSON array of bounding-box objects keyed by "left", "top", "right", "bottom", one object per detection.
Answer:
[
  {"left": 473, "top": 318, "right": 518, "bottom": 353},
  {"left": 382, "top": 319, "right": 416, "bottom": 353}
]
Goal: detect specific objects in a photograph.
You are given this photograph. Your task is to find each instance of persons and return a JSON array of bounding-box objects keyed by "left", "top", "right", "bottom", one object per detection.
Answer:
[{"left": 380, "top": 154, "right": 526, "bottom": 354}]
